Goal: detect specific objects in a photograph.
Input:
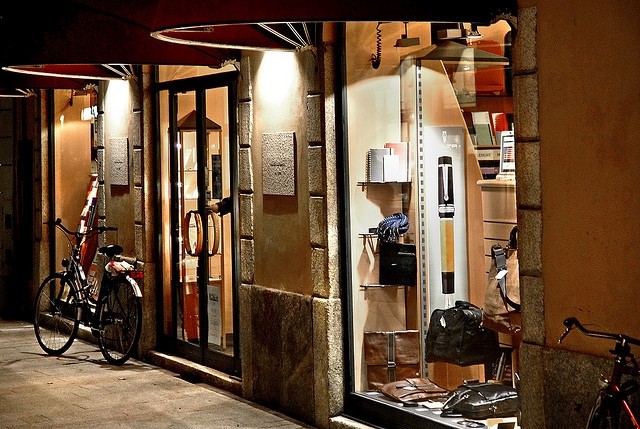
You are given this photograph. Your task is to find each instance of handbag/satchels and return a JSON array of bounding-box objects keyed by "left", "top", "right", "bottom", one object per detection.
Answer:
[
  {"left": 426, "top": 301, "right": 495, "bottom": 366},
  {"left": 378, "top": 377, "right": 451, "bottom": 402},
  {"left": 441, "top": 380, "right": 519, "bottom": 419},
  {"left": 364, "top": 330, "right": 420, "bottom": 390},
  {"left": 483, "top": 226, "right": 522, "bottom": 336}
]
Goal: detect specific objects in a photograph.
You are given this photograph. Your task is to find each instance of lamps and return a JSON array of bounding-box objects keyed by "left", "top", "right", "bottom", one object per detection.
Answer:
[{"left": 467, "top": 23, "right": 482, "bottom": 39}]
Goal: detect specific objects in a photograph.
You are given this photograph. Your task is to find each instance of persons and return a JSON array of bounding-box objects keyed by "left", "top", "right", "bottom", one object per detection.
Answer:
[{"left": 204, "top": 194, "right": 233, "bottom": 216}]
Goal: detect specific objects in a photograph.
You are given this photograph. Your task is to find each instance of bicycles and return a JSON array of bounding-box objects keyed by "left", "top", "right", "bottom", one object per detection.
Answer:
[
  {"left": 557, "top": 317, "right": 640, "bottom": 429},
  {"left": 32, "top": 217, "right": 144, "bottom": 365}
]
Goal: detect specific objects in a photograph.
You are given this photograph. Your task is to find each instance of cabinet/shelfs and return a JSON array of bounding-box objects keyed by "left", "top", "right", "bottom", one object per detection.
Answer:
[{"left": 356, "top": 181, "right": 418, "bottom": 336}]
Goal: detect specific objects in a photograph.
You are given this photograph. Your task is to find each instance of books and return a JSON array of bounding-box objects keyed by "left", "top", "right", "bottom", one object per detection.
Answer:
[{"left": 366, "top": 142, "right": 410, "bottom": 185}]
